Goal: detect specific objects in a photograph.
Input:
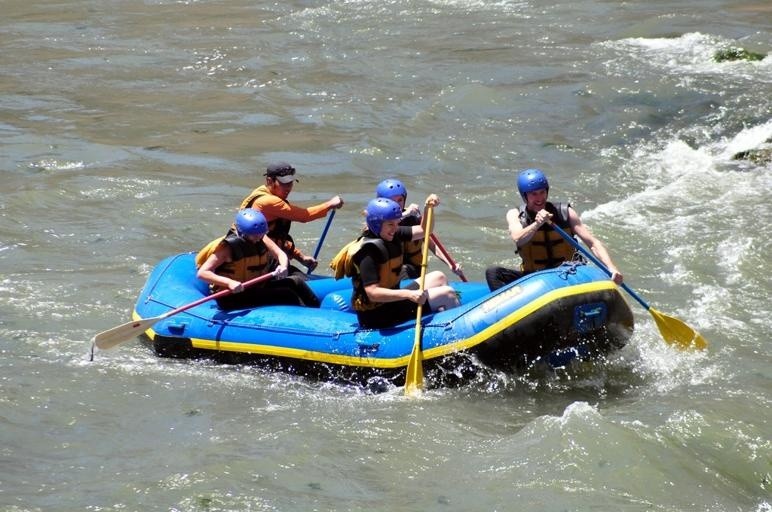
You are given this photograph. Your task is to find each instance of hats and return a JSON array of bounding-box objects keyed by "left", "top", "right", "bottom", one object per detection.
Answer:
[{"left": 267, "top": 162, "right": 301, "bottom": 185}]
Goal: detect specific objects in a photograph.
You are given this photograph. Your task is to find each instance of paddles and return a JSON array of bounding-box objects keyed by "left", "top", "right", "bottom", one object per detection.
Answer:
[
  {"left": 404, "top": 201, "right": 436, "bottom": 402},
  {"left": 94, "top": 271, "right": 278, "bottom": 350},
  {"left": 544, "top": 216, "right": 709, "bottom": 356}
]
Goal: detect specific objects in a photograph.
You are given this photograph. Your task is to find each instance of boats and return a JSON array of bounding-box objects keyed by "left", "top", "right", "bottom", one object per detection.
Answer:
[{"left": 128, "top": 250, "right": 638, "bottom": 397}]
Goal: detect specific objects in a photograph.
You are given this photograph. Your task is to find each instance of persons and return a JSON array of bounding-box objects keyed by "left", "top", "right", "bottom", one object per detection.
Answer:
[
  {"left": 484, "top": 167, "right": 623, "bottom": 292},
  {"left": 365, "top": 177, "right": 463, "bottom": 280},
  {"left": 332, "top": 194, "right": 462, "bottom": 329},
  {"left": 196, "top": 208, "right": 321, "bottom": 313},
  {"left": 227, "top": 159, "right": 344, "bottom": 281}
]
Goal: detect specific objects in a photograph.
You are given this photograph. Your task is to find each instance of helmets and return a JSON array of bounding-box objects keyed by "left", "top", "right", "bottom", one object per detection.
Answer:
[
  {"left": 237, "top": 210, "right": 266, "bottom": 234},
  {"left": 517, "top": 170, "right": 549, "bottom": 202},
  {"left": 366, "top": 199, "right": 402, "bottom": 234},
  {"left": 378, "top": 180, "right": 407, "bottom": 199}
]
